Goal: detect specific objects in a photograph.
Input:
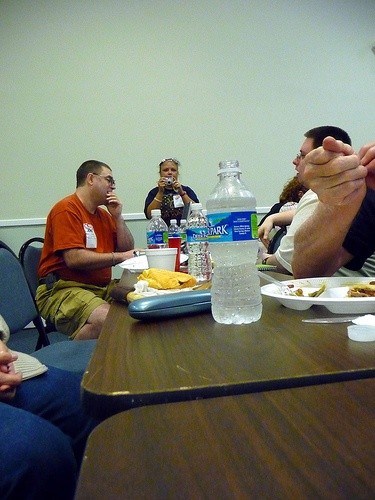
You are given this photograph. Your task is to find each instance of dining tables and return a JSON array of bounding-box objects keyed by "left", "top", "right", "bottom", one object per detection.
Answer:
[{"left": 74, "top": 254, "right": 375, "bottom": 500}]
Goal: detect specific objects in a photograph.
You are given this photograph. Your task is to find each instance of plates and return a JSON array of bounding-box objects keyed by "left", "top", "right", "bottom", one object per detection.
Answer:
[
  {"left": 121, "top": 254, "right": 190, "bottom": 273},
  {"left": 260, "top": 276, "right": 375, "bottom": 314}
]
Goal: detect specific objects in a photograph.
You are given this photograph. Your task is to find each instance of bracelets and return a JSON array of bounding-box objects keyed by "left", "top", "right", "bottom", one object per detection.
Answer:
[
  {"left": 111, "top": 251, "right": 116, "bottom": 266},
  {"left": 154, "top": 197, "right": 163, "bottom": 203},
  {"left": 180, "top": 191, "right": 186, "bottom": 197}
]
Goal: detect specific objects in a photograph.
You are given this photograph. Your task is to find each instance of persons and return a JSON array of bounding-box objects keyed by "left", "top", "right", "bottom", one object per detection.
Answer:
[
  {"left": 0, "top": 314, "right": 115, "bottom": 500},
  {"left": 144, "top": 158, "right": 199, "bottom": 228},
  {"left": 256, "top": 125, "right": 375, "bottom": 280},
  {"left": 35, "top": 160, "right": 147, "bottom": 342}
]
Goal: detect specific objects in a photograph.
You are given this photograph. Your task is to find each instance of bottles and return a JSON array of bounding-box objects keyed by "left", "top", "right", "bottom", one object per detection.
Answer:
[
  {"left": 180, "top": 219, "right": 187, "bottom": 233},
  {"left": 146, "top": 209, "right": 169, "bottom": 249},
  {"left": 168, "top": 219, "right": 179, "bottom": 233},
  {"left": 185, "top": 203, "right": 213, "bottom": 282},
  {"left": 206, "top": 160, "right": 263, "bottom": 325}
]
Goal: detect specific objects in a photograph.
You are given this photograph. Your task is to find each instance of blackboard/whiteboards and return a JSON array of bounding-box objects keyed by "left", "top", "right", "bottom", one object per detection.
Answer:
[{"left": 1, "top": 1, "right": 375, "bottom": 227}]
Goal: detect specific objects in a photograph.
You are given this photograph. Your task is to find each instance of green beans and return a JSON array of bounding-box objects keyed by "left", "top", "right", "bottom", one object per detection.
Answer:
[{"left": 309, "top": 285, "right": 326, "bottom": 296}]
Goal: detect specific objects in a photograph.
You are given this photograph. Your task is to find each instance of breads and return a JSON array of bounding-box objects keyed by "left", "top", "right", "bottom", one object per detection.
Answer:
[{"left": 347, "top": 284, "right": 375, "bottom": 297}]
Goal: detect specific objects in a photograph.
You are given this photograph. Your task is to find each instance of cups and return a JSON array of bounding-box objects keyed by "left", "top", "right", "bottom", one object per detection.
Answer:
[
  {"left": 168, "top": 234, "right": 182, "bottom": 272},
  {"left": 146, "top": 248, "right": 178, "bottom": 271}
]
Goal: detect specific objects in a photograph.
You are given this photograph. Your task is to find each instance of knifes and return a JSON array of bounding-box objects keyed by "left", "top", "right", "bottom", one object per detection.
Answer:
[{"left": 301, "top": 317, "right": 359, "bottom": 324}]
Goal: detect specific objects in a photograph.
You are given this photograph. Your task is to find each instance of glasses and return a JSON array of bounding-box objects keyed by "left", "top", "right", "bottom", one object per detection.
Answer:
[
  {"left": 159, "top": 157, "right": 181, "bottom": 167},
  {"left": 88, "top": 172, "right": 115, "bottom": 186}
]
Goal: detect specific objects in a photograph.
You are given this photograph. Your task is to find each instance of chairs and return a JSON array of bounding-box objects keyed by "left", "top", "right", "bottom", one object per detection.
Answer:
[{"left": 0, "top": 237, "right": 102, "bottom": 380}]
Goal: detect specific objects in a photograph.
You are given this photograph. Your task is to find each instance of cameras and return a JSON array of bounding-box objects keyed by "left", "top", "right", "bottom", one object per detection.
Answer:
[{"left": 163, "top": 177, "right": 175, "bottom": 186}]
